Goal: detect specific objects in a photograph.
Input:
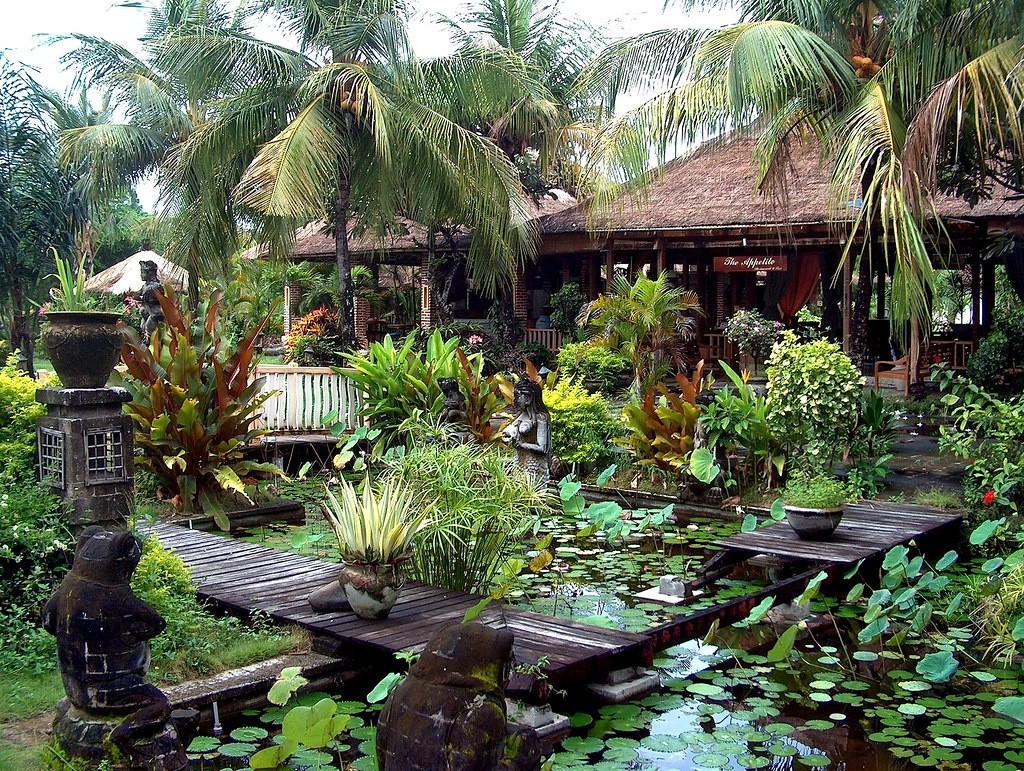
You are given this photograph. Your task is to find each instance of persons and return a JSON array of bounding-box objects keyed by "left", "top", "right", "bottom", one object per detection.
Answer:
[
  {"left": 132, "top": 260, "right": 165, "bottom": 351},
  {"left": 505, "top": 375, "right": 553, "bottom": 491}
]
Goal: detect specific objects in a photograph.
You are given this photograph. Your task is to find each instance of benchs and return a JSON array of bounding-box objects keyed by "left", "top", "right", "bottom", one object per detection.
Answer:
[
  {"left": 672, "top": 343, "right": 716, "bottom": 374},
  {"left": 874, "top": 343, "right": 953, "bottom": 396}
]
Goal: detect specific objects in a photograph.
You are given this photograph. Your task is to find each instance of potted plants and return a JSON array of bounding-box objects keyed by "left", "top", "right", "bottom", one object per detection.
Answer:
[
  {"left": 723, "top": 308, "right": 786, "bottom": 397},
  {"left": 41, "top": 248, "right": 124, "bottom": 388},
  {"left": 323, "top": 470, "right": 442, "bottom": 621},
  {"left": 782, "top": 470, "right": 848, "bottom": 540}
]
